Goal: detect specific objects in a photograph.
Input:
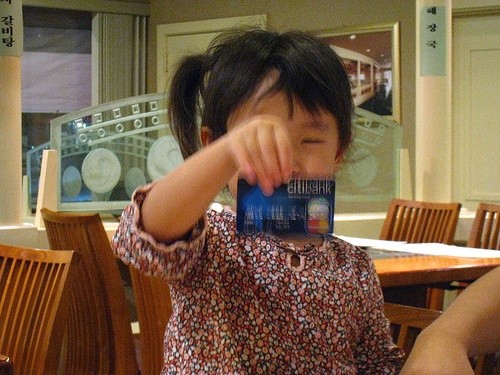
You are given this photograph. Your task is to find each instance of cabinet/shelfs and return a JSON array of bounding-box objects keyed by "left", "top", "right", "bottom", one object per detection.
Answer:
[{"left": 330, "top": 44, "right": 380, "bottom": 106}]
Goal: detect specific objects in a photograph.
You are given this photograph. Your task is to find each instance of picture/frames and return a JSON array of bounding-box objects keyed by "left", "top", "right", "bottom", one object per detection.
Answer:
[{"left": 307, "top": 20, "right": 401, "bottom": 128}]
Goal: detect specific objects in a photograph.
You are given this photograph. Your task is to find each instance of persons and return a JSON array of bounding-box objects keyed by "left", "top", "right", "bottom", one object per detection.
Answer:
[
  {"left": 400, "top": 264, "right": 500, "bottom": 375},
  {"left": 109, "top": 26, "right": 405, "bottom": 375}
]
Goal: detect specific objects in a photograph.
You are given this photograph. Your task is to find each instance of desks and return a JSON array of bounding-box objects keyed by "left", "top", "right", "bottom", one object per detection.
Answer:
[{"left": 103, "top": 231, "right": 500, "bottom": 287}]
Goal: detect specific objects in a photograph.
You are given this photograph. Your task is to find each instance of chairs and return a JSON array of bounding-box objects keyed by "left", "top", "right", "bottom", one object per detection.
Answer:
[
  {"left": 40, "top": 208, "right": 173, "bottom": 375},
  {"left": 0, "top": 244, "right": 81, "bottom": 375},
  {"left": 378, "top": 198, "right": 500, "bottom": 375}
]
